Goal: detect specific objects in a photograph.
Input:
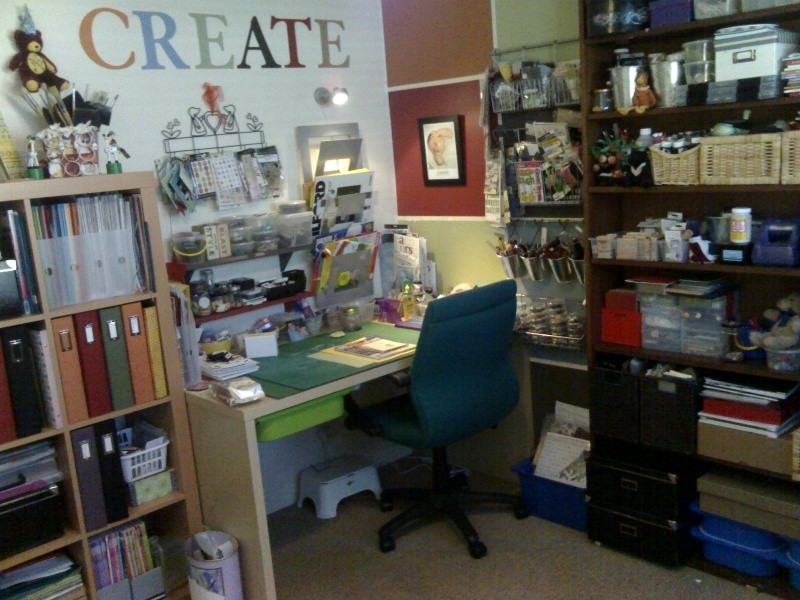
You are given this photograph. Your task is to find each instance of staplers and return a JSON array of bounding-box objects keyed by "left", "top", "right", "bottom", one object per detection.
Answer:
[{"left": 255, "top": 318, "right": 271, "bottom": 332}]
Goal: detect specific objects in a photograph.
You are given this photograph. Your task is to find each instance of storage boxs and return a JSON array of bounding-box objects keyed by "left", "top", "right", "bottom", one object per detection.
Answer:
[
  {"left": 696, "top": 420, "right": 793, "bottom": 476},
  {"left": 696, "top": 469, "right": 800, "bottom": 543},
  {"left": 511, "top": 452, "right": 588, "bottom": 533},
  {"left": 599, "top": 307, "right": 643, "bottom": 348}
]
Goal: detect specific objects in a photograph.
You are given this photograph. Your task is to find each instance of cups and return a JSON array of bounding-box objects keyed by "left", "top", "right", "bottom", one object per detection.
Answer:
[
  {"left": 496, "top": 244, "right": 584, "bottom": 286},
  {"left": 302, "top": 313, "right": 323, "bottom": 333},
  {"left": 592, "top": 40, "right": 715, "bottom": 113},
  {"left": 325, "top": 311, "right": 343, "bottom": 329},
  {"left": 708, "top": 217, "right": 730, "bottom": 243},
  {"left": 346, "top": 298, "right": 376, "bottom": 324}
]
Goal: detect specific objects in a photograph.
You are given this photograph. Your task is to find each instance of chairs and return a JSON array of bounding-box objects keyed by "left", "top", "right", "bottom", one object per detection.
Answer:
[{"left": 342, "top": 279, "right": 519, "bottom": 560}]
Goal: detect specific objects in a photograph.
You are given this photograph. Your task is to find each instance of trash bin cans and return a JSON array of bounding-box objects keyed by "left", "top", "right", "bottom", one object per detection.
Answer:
[{"left": 183, "top": 530, "right": 242, "bottom": 600}]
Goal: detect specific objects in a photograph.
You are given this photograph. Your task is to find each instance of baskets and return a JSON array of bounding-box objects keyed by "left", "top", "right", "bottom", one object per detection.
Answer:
[{"left": 650, "top": 131, "right": 800, "bottom": 185}]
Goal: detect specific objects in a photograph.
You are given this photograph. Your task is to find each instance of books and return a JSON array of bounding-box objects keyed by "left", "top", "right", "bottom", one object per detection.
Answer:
[
  {"left": 0, "top": 193, "right": 171, "bottom": 600},
  {"left": 698, "top": 375, "right": 800, "bottom": 439},
  {"left": 781, "top": 53, "right": 800, "bottom": 98}
]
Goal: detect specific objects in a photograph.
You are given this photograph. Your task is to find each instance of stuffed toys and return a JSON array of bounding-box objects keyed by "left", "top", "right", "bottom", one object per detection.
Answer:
[
  {"left": 9, "top": 6, "right": 71, "bottom": 93},
  {"left": 749, "top": 293, "right": 800, "bottom": 349},
  {"left": 615, "top": 70, "right": 656, "bottom": 115}
]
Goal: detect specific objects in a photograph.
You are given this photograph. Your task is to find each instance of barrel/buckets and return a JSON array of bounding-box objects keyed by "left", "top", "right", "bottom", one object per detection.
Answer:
[{"left": 171, "top": 231, "right": 209, "bottom": 268}]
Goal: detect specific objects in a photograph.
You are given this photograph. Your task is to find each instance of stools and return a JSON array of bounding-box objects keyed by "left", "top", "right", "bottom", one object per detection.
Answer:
[{"left": 297, "top": 453, "right": 384, "bottom": 519}]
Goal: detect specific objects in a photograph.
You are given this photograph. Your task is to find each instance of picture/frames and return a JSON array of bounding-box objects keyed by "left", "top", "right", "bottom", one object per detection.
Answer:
[{"left": 416, "top": 113, "right": 467, "bottom": 187}]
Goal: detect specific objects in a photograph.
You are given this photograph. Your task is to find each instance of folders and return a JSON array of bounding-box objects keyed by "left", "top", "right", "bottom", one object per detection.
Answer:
[
  {"left": 94, "top": 420, "right": 128, "bottom": 522},
  {"left": 99, "top": 307, "right": 134, "bottom": 410},
  {"left": 51, "top": 317, "right": 90, "bottom": 424},
  {"left": 69, "top": 427, "right": 107, "bottom": 531},
  {"left": 75, "top": 310, "right": 112, "bottom": 419},
  {"left": 121, "top": 302, "right": 155, "bottom": 404}
]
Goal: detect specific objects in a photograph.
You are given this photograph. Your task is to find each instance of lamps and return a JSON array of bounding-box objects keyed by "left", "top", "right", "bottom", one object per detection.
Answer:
[{"left": 313, "top": 84, "right": 350, "bottom": 107}]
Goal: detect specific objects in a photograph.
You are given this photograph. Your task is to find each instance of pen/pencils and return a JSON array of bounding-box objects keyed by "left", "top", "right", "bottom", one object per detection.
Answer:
[{"left": 387, "top": 289, "right": 403, "bottom": 300}]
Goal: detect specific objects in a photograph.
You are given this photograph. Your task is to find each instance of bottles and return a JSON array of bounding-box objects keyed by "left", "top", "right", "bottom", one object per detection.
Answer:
[
  {"left": 191, "top": 269, "right": 243, "bottom": 316},
  {"left": 517, "top": 297, "right": 585, "bottom": 347},
  {"left": 631, "top": 127, "right": 704, "bottom": 188},
  {"left": 730, "top": 207, "right": 752, "bottom": 243},
  {"left": 397, "top": 284, "right": 415, "bottom": 321},
  {"left": 343, "top": 307, "right": 362, "bottom": 333}
]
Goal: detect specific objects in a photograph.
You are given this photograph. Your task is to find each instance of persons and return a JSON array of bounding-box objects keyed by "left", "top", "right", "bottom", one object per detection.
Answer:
[{"left": 427, "top": 127, "right": 455, "bottom": 169}]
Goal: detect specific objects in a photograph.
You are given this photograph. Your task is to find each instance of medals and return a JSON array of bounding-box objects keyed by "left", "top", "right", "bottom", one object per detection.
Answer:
[{"left": 336, "top": 334, "right": 416, "bottom": 358}]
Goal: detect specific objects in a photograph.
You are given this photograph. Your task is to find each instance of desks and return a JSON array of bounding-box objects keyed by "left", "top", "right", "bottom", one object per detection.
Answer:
[{"left": 183, "top": 308, "right": 428, "bottom": 600}]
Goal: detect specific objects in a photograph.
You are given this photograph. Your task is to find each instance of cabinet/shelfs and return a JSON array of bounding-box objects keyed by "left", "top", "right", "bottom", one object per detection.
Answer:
[
  {"left": 482, "top": 0, "right": 800, "bottom": 600},
  {"left": 0, "top": 128, "right": 315, "bottom": 600}
]
